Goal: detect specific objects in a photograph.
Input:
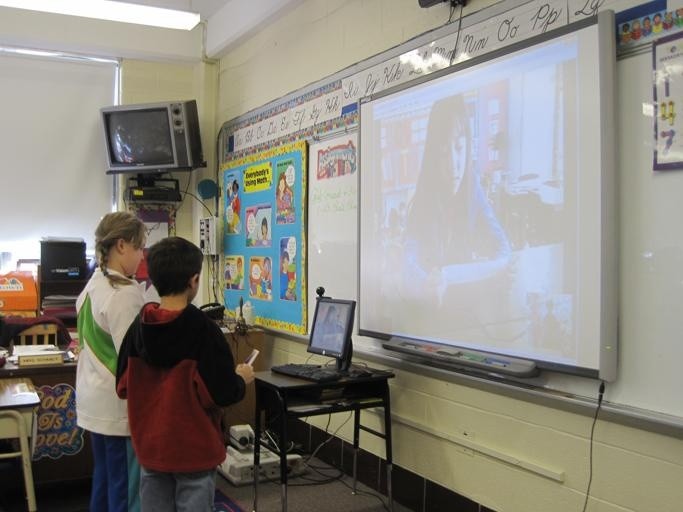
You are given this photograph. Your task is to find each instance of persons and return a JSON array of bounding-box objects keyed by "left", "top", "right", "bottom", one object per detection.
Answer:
[
  {"left": 315, "top": 305, "right": 344, "bottom": 353},
  {"left": 222, "top": 171, "right": 297, "bottom": 302},
  {"left": 73, "top": 211, "right": 148, "bottom": 512},
  {"left": 399, "top": 93, "right": 513, "bottom": 299},
  {"left": 115, "top": 236, "right": 257, "bottom": 512}
]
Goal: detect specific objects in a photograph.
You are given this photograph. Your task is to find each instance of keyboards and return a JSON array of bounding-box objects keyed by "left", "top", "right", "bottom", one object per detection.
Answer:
[{"left": 271, "top": 363, "right": 340, "bottom": 382}]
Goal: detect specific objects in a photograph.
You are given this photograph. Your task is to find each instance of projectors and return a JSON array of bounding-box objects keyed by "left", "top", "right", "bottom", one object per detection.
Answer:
[{"left": 217, "top": 443, "right": 282, "bottom": 486}]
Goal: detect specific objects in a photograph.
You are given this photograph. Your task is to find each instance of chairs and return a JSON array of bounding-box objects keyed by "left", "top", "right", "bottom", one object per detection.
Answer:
[{"left": 0, "top": 317, "right": 60, "bottom": 346}]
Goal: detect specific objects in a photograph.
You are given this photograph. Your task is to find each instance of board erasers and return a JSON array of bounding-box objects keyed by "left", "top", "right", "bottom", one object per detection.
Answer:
[{"left": 436, "top": 347, "right": 461, "bottom": 357}]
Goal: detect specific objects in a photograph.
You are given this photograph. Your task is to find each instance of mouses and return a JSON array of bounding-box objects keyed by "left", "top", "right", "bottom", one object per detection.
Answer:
[{"left": 350, "top": 367, "right": 371, "bottom": 377}]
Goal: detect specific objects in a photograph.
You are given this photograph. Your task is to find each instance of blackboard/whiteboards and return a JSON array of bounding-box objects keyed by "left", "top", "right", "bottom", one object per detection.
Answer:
[
  {"left": 215, "top": 0, "right": 683, "bottom": 439},
  {"left": 356, "top": 10, "right": 620, "bottom": 383}
]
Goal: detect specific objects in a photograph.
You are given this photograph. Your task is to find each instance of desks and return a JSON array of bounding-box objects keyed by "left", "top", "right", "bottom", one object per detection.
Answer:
[
  {"left": 0, "top": 377, "right": 41, "bottom": 512},
  {"left": 252, "top": 362, "right": 396, "bottom": 512}
]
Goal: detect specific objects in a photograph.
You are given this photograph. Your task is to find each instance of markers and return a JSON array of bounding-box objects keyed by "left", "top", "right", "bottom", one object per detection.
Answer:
[{"left": 463, "top": 354, "right": 510, "bottom": 367}]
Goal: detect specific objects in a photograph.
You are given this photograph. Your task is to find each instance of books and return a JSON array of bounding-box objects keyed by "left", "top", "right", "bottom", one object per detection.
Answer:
[
  {"left": 12, "top": 345, "right": 64, "bottom": 364},
  {"left": 41, "top": 294, "right": 79, "bottom": 308},
  {"left": 243, "top": 347, "right": 260, "bottom": 366}
]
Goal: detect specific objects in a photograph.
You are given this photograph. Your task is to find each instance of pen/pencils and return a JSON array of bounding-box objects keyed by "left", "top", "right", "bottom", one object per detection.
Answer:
[{"left": 402, "top": 344, "right": 436, "bottom": 354}]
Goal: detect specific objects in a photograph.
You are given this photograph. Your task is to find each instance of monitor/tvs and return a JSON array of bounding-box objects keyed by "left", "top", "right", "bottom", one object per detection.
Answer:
[
  {"left": 306, "top": 298, "right": 357, "bottom": 373},
  {"left": 99, "top": 100, "right": 204, "bottom": 169}
]
